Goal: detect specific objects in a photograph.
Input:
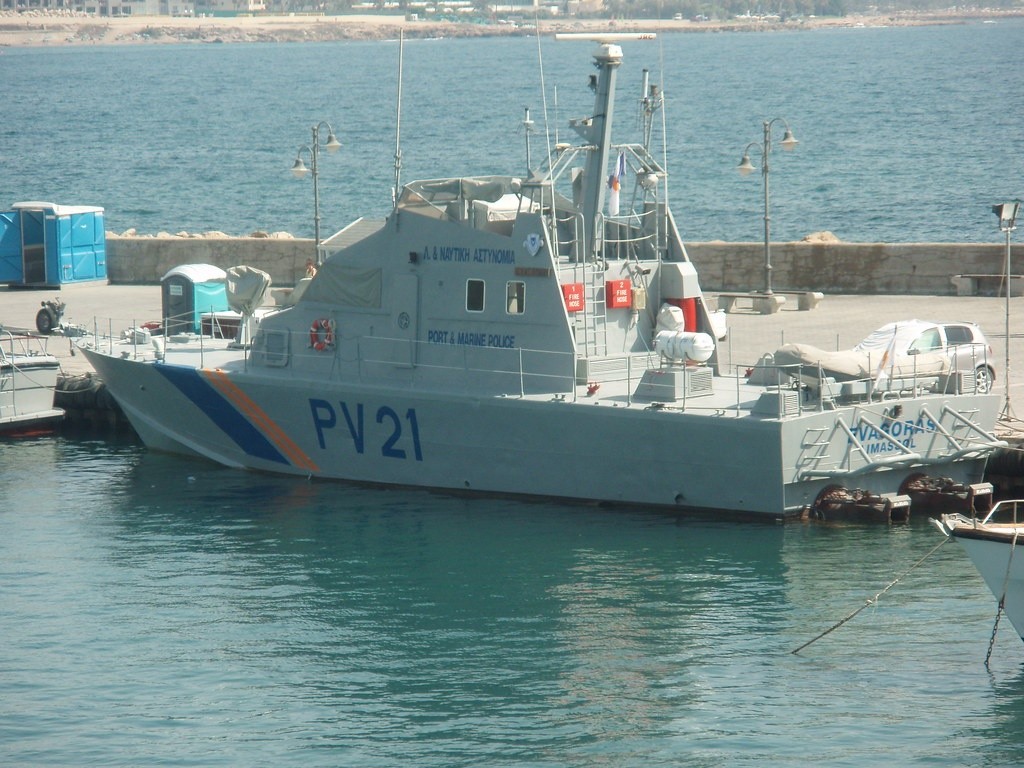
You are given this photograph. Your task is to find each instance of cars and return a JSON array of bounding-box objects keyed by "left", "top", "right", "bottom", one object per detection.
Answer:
[{"left": 851, "top": 322, "right": 996, "bottom": 393}]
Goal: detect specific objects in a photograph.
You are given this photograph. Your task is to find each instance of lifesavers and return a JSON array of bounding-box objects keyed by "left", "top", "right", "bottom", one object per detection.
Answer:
[{"left": 311, "top": 320, "right": 332, "bottom": 350}]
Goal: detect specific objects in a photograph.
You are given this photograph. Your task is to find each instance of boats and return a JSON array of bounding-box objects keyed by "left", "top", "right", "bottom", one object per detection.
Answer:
[{"left": 59, "top": 40, "right": 1016, "bottom": 519}]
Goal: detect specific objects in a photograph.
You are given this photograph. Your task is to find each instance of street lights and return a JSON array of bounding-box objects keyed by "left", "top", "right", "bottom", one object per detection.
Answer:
[
  {"left": 737, "top": 116, "right": 800, "bottom": 296},
  {"left": 991, "top": 202, "right": 1021, "bottom": 421},
  {"left": 291, "top": 121, "right": 344, "bottom": 269}
]
